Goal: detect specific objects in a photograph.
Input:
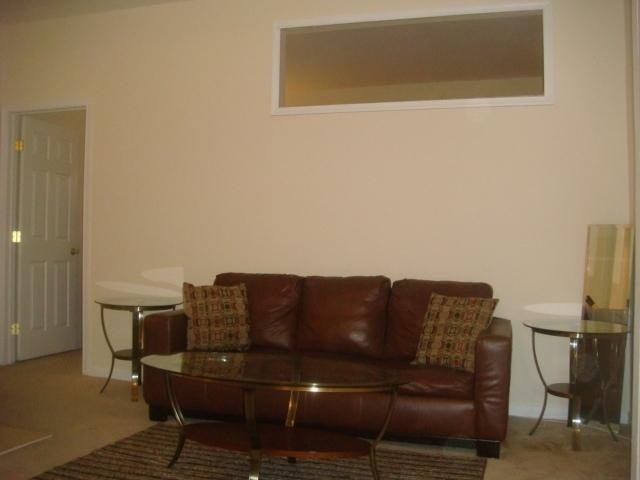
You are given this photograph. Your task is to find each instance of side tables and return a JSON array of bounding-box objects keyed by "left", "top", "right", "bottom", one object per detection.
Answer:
[
  {"left": 93, "top": 294, "right": 185, "bottom": 402},
  {"left": 522, "top": 319, "right": 630, "bottom": 451}
]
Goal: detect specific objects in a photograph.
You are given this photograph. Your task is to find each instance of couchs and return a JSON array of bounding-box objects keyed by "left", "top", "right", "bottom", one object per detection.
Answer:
[{"left": 144, "top": 273, "right": 511, "bottom": 458}]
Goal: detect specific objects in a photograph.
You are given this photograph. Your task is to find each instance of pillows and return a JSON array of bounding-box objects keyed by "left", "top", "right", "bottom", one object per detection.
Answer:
[
  {"left": 411, "top": 293, "right": 497, "bottom": 374},
  {"left": 183, "top": 282, "right": 254, "bottom": 353}
]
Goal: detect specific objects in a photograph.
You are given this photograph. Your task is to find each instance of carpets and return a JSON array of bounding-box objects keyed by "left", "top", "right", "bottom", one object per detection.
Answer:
[{"left": 30, "top": 421, "right": 488, "bottom": 480}]
